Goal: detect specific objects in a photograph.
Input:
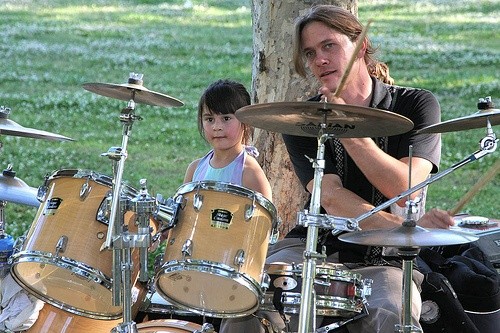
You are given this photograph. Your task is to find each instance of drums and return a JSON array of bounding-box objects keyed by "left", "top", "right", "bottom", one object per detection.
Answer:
[
  {"left": 9, "top": 168, "right": 161, "bottom": 321},
  {"left": 156, "top": 180, "right": 279, "bottom": 320},
  {"left": 24, "top": 270, "right": 219, "bottom": 333},
  {"left": 256, "top": 262, "right": 368, "bottom": 319}
]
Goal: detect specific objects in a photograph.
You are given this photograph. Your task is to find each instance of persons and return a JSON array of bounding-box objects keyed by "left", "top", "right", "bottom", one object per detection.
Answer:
[
  {"left": 149, "top": 80, "right": 272, "bottom": 252},
  {"left": 219, "top": 5, "right": 456, "bottom": 333}
]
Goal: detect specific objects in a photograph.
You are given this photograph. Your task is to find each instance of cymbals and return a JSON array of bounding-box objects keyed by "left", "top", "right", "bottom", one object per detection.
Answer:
[
  {"left": 337, "top": 225, "right": 480, "bottom": 246},
  {"left": 82, "top": 82, "right": 184, "bottom": 108},
  {"left": 235, "top": 102, "right": 414, "bottom": 138},
  {"left": 0, "top": 117, "right": 76, "bottom": 142},
  {"left": 0, "top": 174, "right": 39, "bottom": 209},
  {"left": 417, "top": 107, "right": 500, "bottom": 135}
]
